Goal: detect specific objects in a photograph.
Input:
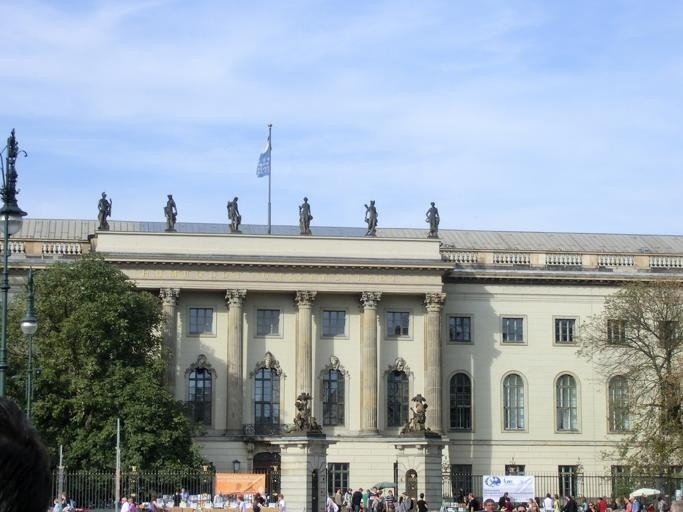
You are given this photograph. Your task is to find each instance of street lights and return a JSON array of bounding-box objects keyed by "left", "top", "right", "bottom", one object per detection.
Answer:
[
  {"left": 0, "top": 126, "right": 28, "bottom": 398},
  {"left": 20, "top": 264, "right": 38, "bottom": 416}
]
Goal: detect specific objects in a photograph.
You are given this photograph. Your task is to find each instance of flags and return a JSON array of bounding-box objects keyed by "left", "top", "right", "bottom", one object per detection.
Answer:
[{"left": 257, "top": 132, "right": 270, "bottom": 177}]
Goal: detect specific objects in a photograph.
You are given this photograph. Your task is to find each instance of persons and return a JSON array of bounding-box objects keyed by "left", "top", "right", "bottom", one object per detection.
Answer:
[
  {"left": 299, "top": 196, "right": 313, "bottom": 235},
  {"left": 364, "top": 199, "right": 378, "bottom": 237},
  {"left": 227, "top": 196, "right": 242, "bottom": 235},
  {"left": 163, "top": 195, "right": 177, "bottom": 232},
  {"left": 425, "top": 202, "right": 440, "bottom": 240},
  {"left": 97, "top": 192, "right": 111, "bottom": 233}
]
